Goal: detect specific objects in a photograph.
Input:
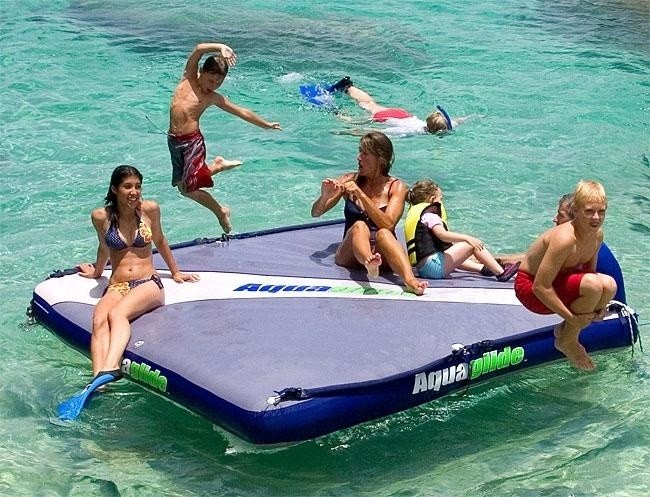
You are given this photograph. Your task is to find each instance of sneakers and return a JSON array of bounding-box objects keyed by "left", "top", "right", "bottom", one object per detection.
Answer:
[
  {"left": 497, "top": 260, "right": 521, "bottom": 281},
  {"left": 483, "top": 259, "right": 502, "bottom": 275}
]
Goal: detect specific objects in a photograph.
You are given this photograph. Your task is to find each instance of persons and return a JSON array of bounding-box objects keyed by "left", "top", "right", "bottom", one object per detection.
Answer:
[
  {"left": 167, "top": 44, "right": 283, "bottom": 234},
  {"left": 311, "top": 132, "right": 429, "bottom": 296},
  {"left": 309, "top": 76, "right": 467, "bottom": 138},
  {"left": 404, "top": 180, "right": 522, "bottom": 282},
  {"left": 494, "top": 193, "right": 627, "bottom": 308},
  {"left": 75, "top": 166, "right": 200, "bottom": 394},
  {"left": 514, "top": 181, "right": 618, "bottom": 371}
]
